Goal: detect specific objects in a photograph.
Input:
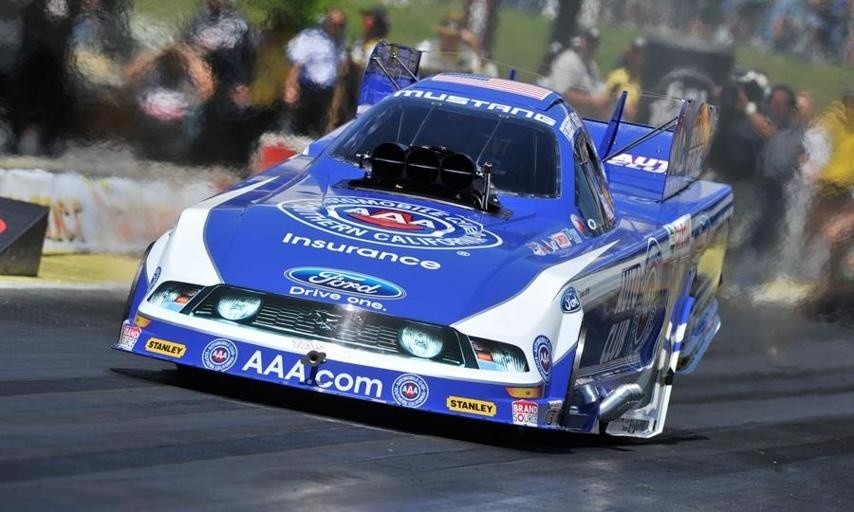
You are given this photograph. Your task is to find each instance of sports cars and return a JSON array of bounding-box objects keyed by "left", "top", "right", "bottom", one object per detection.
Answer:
[{"left": 109, "top": 41, "right": 734, "bottom": 442}]
